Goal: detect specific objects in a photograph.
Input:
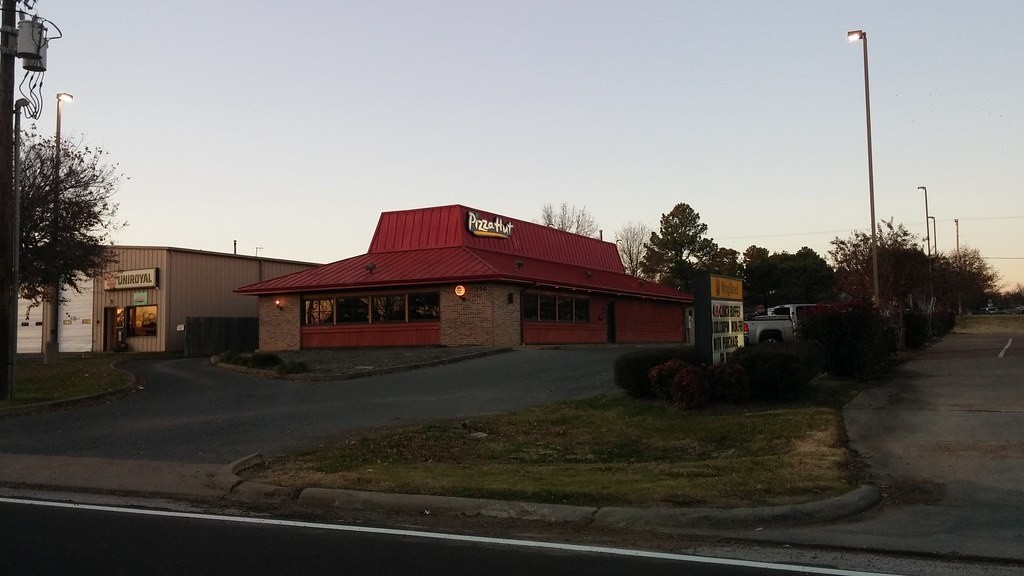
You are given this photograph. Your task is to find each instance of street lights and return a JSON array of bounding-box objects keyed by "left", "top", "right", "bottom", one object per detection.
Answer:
[
  {"left": 928, "top": 214, "right": 936, "bottom": 255},
  {"left": 12, "top": 97, "right": 28, "bottom": 403},
  {"left": 43, "top": 92, "right": 74, "bottom": 367},
  {"left": 918, "top": 187, "right": 933, "bottom": 300},
  {"left": 953, "top": 219, "right": 961, "bottom": 318},
  {"left": 847, "top": 28, "right": 878, "bottom": 310}
]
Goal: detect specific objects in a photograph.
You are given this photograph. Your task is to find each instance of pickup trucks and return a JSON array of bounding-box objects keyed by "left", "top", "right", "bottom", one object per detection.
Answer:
[{"left": 743, "top": 302, "right": 840, "bottom": 346}]
